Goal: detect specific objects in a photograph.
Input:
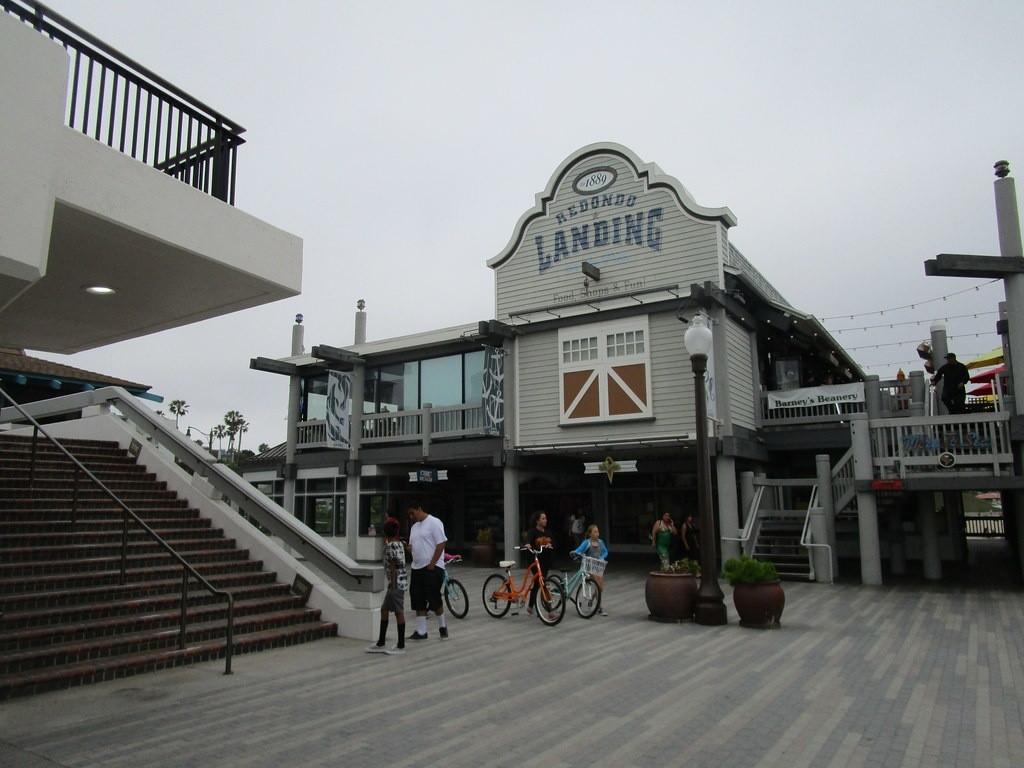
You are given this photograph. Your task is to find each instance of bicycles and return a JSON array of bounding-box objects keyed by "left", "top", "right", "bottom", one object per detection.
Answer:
[
  {"left": 425, "top": 555, "right": 469, "bottom": 618},
  {"left": 541, "top": 552, "right": 608, "bottom": 619},
  {"left": 482, "top": 546, "right": 566, "bottom": 627}
]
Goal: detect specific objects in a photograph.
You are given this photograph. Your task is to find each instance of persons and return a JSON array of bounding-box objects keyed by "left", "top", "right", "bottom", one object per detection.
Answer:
[
  {"left": 820, "top": 375, "right": 833, "bottom": 386},
  {"left": 401, "top": 498, "right": 449, "bottom": 642},
  {"left": 682, "top": 513, "right": 699, "bottom": 559},
  {"left": 652, "top": 510, "right": 677, "bottom": 572},
  {"left": 524, "top": 510, "right": 558, "bottom": 620},
  {"left": 570, "top": 524, "right": 608, "bottom": 617},
  {"left": 365, "top": 517, "right": 407, "bottom": 655},
  {"left": 930, "top": 353, "right": 970, "bottom": 414}
]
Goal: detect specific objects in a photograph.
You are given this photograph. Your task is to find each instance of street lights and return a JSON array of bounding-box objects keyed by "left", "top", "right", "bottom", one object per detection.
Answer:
[{"left": 683, "top": 313, "right": 727, "bottom": 627}]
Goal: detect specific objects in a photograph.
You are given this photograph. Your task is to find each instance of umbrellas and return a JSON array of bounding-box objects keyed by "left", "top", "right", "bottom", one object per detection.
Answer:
[
  {"left": 970, "top": 364, "right": 1007, "bottom": 384},
  {"left": 975, "top": 492, "right": 1001, "bottom": 500},
  {"left": 966, "top": 384, "right": 1008, "bottom": 396},
  {"left": 965, "top": 347, "right": 1005, "bottom": 369}
]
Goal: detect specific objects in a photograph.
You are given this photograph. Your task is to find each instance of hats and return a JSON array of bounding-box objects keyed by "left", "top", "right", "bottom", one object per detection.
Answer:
[{"left": 943, "top": 353, "right": 956, "bottom": 359}]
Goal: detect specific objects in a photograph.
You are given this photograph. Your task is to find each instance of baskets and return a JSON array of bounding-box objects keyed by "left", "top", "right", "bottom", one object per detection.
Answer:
[
  {"left": 541, "top": 550, "right": 562, "bottom": 569},
  {"left": 583, "top": 557, "right": 608, "bottom": 577}
]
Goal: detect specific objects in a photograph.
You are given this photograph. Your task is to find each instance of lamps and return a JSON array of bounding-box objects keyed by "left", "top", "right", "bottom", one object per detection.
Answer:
[
  {"left": 356, "top": 299, "right": 365, "bottom": 312},
  {"left": 295, "top": 313, "right": 304, "bottom": 325}
]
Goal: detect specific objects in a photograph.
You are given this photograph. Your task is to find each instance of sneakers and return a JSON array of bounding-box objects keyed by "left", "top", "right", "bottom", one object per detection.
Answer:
[
  {"left": 438, "top": 625, "right": 448, "bottom": 640},
  {"left": 364, "top": 644, "right": 386, "bottom": 653},
  {"left": 404, "top": 630, "right": 429, "bottom": 642},
  {"left": 385, "top": 646, "right": 406, "bottom": 654}
]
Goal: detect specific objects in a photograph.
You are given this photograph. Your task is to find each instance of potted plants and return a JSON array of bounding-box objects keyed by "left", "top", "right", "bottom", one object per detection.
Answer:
[
  {"left": 722, "top": 553, "right": 786, "bottom": 628},
  {"left": 643, "top": 556, "right": 701, "bottom": 624}
]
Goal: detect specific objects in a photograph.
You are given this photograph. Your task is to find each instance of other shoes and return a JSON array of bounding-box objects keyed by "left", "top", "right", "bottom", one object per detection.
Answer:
[
  {"left": 597, "top": 607, "right": 608, "bottom": 616},
  {"left": 587, "top": 601, "right": 593, "bottom": 610},
  {"left": 526, "top": 607, "right": 536, "bottom": 616},
  {"left": 546, "top": 613, "right": 559, "bottom": 623}
]
